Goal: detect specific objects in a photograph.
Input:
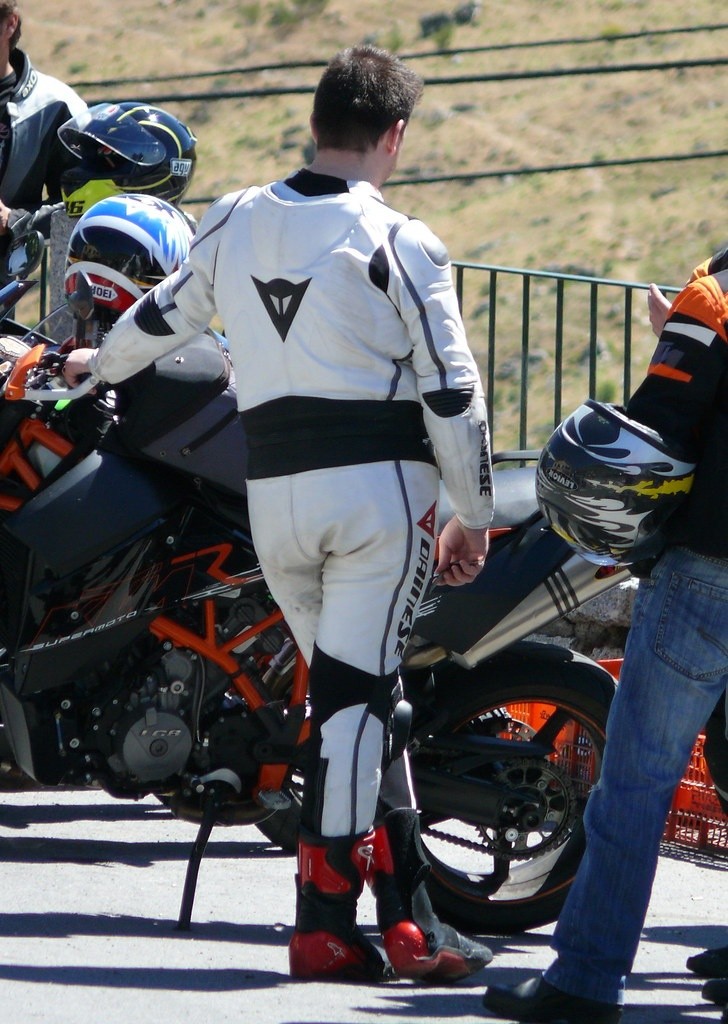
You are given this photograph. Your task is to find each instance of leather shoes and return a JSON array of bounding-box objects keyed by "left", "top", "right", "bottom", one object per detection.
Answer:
[
  {"left": 481, "top": 969, "right": 625, "bottom": 1024},
  {"left": 701, "top": 979, "right": 728, "bottom": 1002}
]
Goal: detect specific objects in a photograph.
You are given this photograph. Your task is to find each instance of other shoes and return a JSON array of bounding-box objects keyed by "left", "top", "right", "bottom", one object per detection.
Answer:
[
  {"left": 721, "top": 1009, "right": 728, "bottom": 1024},
  {"left": 685, "top": 945, "right": 728, "bottom": 978}
]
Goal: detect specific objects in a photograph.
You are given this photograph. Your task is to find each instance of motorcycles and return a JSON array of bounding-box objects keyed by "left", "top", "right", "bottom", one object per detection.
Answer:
[{"left": 1, "top": 108, "right": 630, "bottom": 938}]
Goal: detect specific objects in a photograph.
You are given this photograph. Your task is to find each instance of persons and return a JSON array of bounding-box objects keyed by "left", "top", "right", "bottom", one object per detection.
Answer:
[
  {"left": 482, "top": 243, "right": 728, "bottom": 1023},
  {"left": 61, "top": 0, "right": 493, "bottom": 981},
  {"left": 0, "top": 0, "right": 92, "bottom": 341}
]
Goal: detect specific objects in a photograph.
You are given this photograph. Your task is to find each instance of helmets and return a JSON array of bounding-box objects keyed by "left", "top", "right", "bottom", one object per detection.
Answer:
[
  {"left": 534, "top": 397, "right": 698, "bottom": 567},
  {"left": 62, "top": 193, "right": 200, "bottom": 313},
  {"left": 54, "top": 99, "right": 200, "bottom": 220}
]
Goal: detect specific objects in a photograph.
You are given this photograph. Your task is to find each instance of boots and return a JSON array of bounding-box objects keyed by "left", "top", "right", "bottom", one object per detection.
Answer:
[
  {"left": 288, "top": 816, "right": 386, "bottom": 987},
  {"left": 348, "top": 806, "right": 494, "bottom": 984}
]
